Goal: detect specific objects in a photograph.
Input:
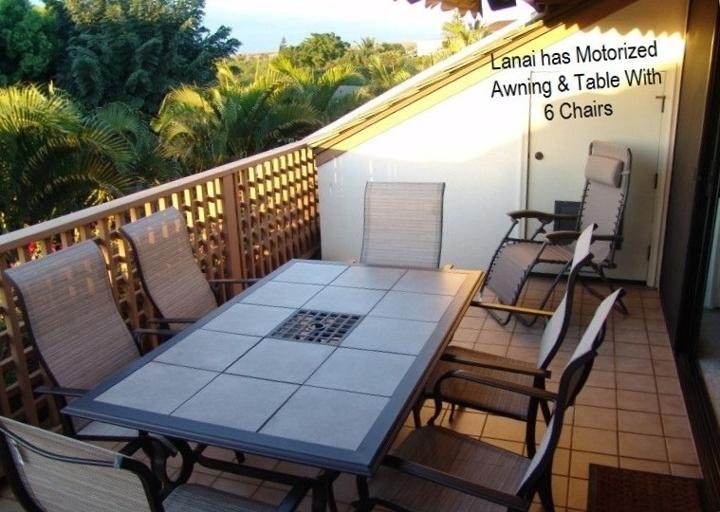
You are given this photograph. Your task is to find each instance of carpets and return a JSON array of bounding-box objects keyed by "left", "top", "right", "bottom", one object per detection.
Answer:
[{"left": 584, "top": 459, "right": 720, "bottom": 512}]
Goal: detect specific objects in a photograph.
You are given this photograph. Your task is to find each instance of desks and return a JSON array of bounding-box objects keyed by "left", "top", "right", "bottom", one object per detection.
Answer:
[{"left": 58, "top": 256, "right": 487, "bottom": 509}]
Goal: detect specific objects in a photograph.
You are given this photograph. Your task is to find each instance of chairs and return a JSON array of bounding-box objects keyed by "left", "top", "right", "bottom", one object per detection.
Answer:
[
  {"left": 1, "top": 408, "right": 315, "bottom": 512},
  {"left": 121, "top": 206, "right": 260, "bottom": 333},
  {"left": 5, "top": 240, "right": 246, "bottom": 489},
  {"left": 406, "top": 224, "right": 599, "bottom": 459},
  {"left": 479, "top": 136, "right": 636, "bottom": 326},
  {"left": 326, "top": 289, "right": 628, "bottom": 509},
  {"left": 359, "top": 178, "right": 446, "bottom": 271}
]
